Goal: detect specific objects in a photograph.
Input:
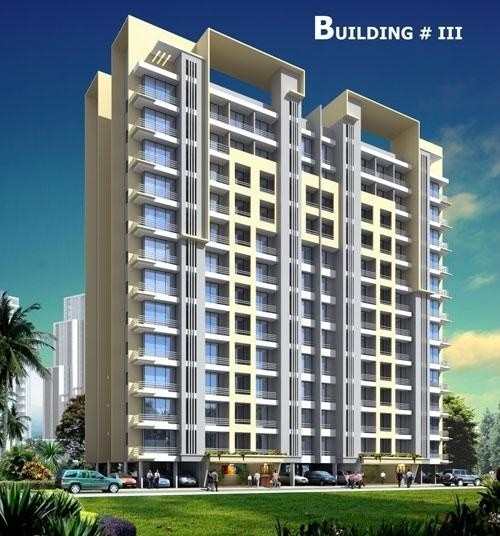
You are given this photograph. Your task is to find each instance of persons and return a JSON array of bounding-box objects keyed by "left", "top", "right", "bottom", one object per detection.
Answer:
[
  {"left": 406, "top": 469, "right": 415, "bottom": 487},
  {"left": 147, "top": 469, "right": 153, "bottom": 488},
  {"left": 395, "top": 469, "right": 403, "bottom": 487},
  {"left": 154, "top": 468, "right": 161, "bottom": 488},
  {"left": 381, "top": 471, "right": 386, "bottom": 485},
  {"left": 212, "top": 469, "right": 219, "bottom": 491},
  {"left": 247, "top": 473, "right": 253, "bottom": 488},
  {"left": 253, "top": 471, "right": 261, "bottom": 488},
  {"left": 345, "top": 471, "right": 365, "bottom": 489},
  {"left": 207, "top": 470, "right": 214, "bottom": 491},
  {"left": 270, "top": 470, "right": 281, "bottom": 487}
]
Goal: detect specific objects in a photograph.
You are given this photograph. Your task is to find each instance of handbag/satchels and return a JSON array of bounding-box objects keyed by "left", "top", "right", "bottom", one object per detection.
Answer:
[{"left": 409, "top": 477, "right": 414, "bottom": 480}]
